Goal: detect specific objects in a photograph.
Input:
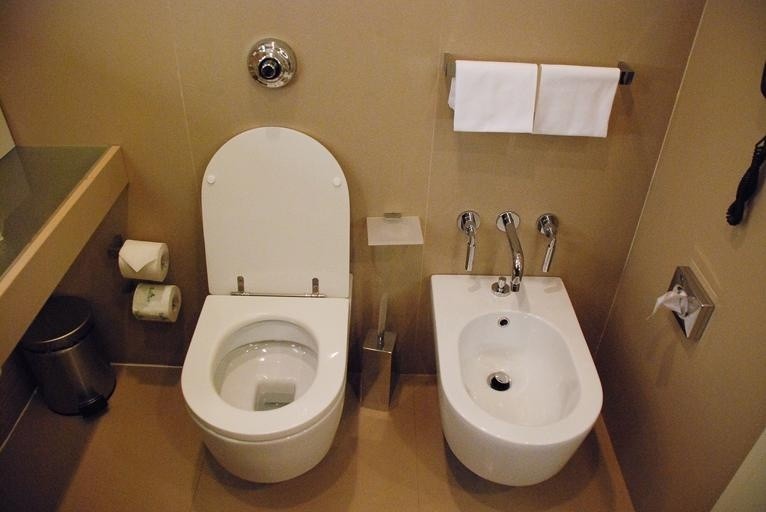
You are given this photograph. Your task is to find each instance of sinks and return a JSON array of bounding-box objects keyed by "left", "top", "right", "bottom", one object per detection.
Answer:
[{"left": 432, "top": 275, "right": 603, "bottom": 489}]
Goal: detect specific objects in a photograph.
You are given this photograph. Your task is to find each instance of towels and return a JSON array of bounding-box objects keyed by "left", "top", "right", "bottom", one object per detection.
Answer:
[
  {"left": 534, "top": 64, "right": 621, "bottom": 138},
  {"left": 448, "top": 60, "right": 539, "bottom": 134}
]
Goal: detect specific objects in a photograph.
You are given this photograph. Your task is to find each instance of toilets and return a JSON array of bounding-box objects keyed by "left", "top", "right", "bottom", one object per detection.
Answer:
[{"left": 181, "top": 127, "right": 354, "bottom": 484}]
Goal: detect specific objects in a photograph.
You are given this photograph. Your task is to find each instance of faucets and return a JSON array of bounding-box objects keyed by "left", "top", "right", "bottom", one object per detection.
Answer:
[{"left": 503, "top": 213, "right": 524, "bottom": 293}]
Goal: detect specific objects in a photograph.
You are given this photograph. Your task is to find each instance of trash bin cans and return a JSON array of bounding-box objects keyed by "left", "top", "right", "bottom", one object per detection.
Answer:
[{"left": 18, "top": 295, "right": 116, "bottom": 419}]
